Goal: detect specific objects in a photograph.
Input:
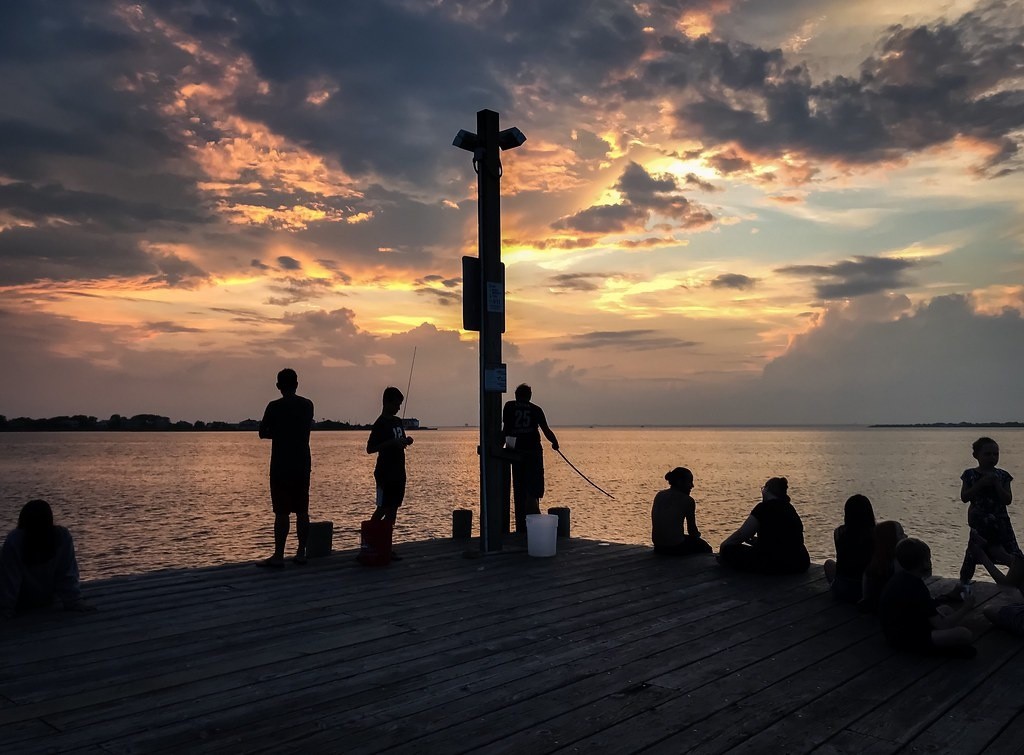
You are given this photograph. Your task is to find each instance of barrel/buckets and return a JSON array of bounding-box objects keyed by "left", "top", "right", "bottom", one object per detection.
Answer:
[
  {"left": 548, "top": 507, "right": 570, "bottom": 540},
  {"left": 306, "top": 522, "right": 332, "bottom": 557},
  {"left": 525, "top": 514, "right": 559, "bottom": 557},
  {"left": 452, "top": 510, "right": 472, "bottom": 544},
  {"left": 360, "top": 520, "right": 393, "bottom": 567}
]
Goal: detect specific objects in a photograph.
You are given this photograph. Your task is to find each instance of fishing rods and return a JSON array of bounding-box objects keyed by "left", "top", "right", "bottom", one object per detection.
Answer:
[
  {"left": 400, "top": 345, "right": 421, "bottom": 419},
  {"left": 556, "top": 447, "right": 618, "bottom": 502}
]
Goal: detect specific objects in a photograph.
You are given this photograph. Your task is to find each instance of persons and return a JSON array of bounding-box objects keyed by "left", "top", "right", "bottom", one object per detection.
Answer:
[
  {"left": 721, "top": 478, "right": 810, "bottom": 577},
  {"left": 1, "top": 500, "right": 98, "bottom": 618},
  {"left": 502, "top": 385, "right": 559, "bottom": 540},
  {"left": 365, "top": 388, "right": 412, "bottom": 559},
  {"left": 255, "top": 370, "right": 313, "bottom": 567},
  {"left": 823, "top": 438, "right": 1023, "bottom": 659},
  {"left": 652, "top": 469, "right": 714, "bottom": 556}
]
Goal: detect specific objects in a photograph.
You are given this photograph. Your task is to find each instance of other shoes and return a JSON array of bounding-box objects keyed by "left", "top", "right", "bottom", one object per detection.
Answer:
[
  {"left": 391, "top": 551, "right": 403, "bottom": 561},
  {"left": 255, "top": 559, "right": 285, "bottom": 568},
  {"left": 294, "top": 556, "right": 307, "bottom": 564}
]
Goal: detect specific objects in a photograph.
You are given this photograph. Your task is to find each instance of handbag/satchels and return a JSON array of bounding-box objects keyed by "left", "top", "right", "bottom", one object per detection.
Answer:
[{"left": 361, "top": 519, "right": 392, "bottom": 566}]
